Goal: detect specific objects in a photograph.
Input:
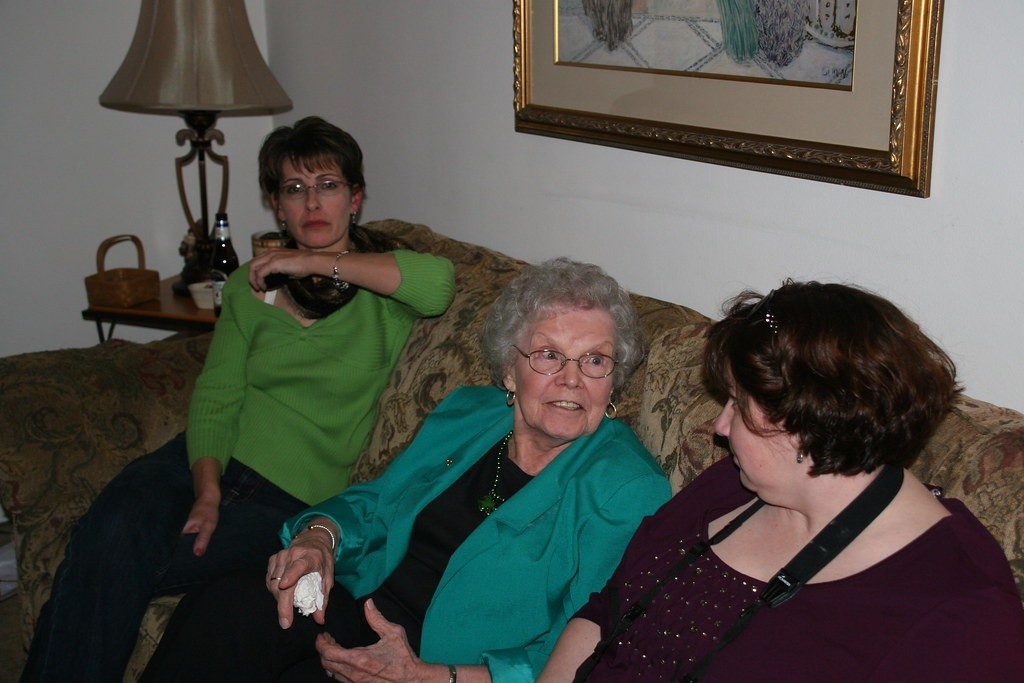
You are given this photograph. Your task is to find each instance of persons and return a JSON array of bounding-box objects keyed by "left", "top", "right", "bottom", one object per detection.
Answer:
[
  {"left": 186, "top": 270, "right": 674, "bottom": 683},
  {"left": 531, "top": 276, "right": 1024, "bottom": 682},
  {"left": 19, "top": 117, "right": 456, "bottom": 683}
]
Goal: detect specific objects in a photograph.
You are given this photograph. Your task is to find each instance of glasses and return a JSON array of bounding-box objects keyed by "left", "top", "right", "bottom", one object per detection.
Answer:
[
  {"left": 511, "top": 342, "right": 621, "bottom": 378},
  {"left": 745, "top": 285, "right": 786, "bottom": 385},
  {"left": 275, "top": 179, "right": 352, "bottom": 198}
]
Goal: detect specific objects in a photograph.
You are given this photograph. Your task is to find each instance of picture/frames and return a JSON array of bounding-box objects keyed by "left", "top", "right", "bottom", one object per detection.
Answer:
[{"left": 512, "top": 0, "right": 944, "bottom": 199}]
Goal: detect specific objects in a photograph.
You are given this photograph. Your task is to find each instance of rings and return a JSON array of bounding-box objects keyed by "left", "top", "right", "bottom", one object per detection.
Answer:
[{"left": 270, "top": 577, "right": 282, "bottom": 581}]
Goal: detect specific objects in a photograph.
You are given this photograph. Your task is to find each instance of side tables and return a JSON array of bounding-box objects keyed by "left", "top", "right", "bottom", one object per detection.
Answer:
[{"left": 82, "top": 272, "right": 220, "bottom": 342}]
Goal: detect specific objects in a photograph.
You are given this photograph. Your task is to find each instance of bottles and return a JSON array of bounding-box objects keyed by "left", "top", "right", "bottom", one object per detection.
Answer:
[{"left": 211, "top": 214, "right": 240, "bottom": 318}]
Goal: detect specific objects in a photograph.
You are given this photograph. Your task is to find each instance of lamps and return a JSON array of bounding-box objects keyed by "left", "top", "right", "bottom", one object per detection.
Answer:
[{"left": 99, "top": 0, "right": 294, "bottom": 281}]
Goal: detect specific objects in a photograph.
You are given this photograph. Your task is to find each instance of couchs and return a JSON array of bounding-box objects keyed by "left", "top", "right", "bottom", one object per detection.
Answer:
[{"left": 1, "top": 219, "right": 1024, "bottom": 683}]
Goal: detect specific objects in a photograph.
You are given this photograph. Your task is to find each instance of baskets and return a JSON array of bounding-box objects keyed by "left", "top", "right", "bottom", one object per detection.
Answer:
[{"left": 85, "top": 232, "right": 161, "bottom": 309}]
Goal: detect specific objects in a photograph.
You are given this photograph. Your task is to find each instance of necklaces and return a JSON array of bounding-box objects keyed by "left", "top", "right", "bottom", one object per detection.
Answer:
[{"left": 478, "top": 430, "right": 521, "bottom": 518}]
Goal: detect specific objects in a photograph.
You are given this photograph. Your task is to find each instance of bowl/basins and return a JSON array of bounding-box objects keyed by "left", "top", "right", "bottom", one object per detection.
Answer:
[{"left": 188, "top": 282, "right": 214, "bottom": 309}]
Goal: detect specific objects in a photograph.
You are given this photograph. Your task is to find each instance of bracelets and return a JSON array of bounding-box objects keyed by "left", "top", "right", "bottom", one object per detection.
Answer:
[
  {"left": 332, "top": 250, "right": 349, "bottom": 287},
  {"left": 448, "top": 664, "right": 456, "bottom": 683},
  {"left": 290, "top": 523, "right": 336, "bottom": 554}
]
